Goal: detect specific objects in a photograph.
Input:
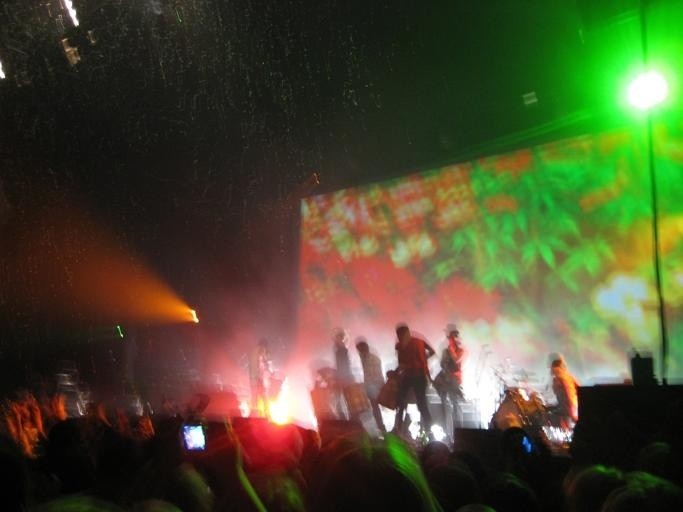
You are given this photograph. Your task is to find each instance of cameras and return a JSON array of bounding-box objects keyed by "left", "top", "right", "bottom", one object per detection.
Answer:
[
  {"left": 522, "top": 435, "right": 534, "bottom": 454},
  {"left": 180, "top": 421, "right": 223, "bottom": 453}
]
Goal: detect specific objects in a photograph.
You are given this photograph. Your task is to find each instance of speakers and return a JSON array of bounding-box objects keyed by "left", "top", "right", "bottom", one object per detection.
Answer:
[
  {"left": 454, "top": 428, "right": 487, "bottom": 452},
  {"left": 319, "top": 421, "right": 374, "bottom": 445}
]
